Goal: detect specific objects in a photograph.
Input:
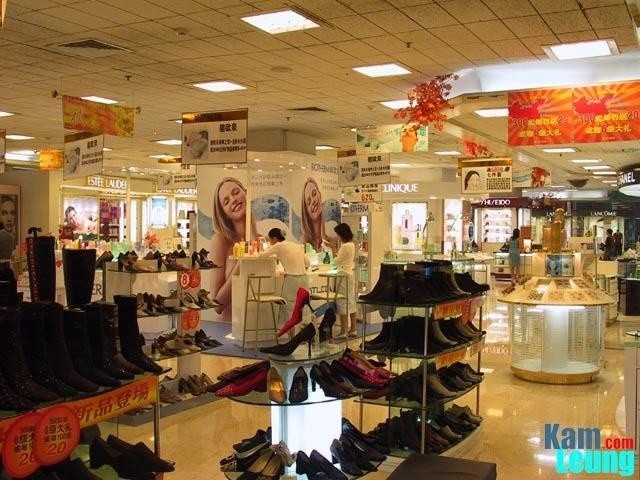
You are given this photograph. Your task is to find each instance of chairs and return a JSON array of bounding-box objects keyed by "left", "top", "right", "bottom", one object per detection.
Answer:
[
  {"left": 310, "top": 272, "right": 349, "bottom": 349},
  {"left": 241, "top": 274, "right": 294, "bottom": 356}
]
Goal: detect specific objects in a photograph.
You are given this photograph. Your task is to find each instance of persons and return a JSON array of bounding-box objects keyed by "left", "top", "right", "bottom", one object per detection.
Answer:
[
  {"left": 64, "top": 206, "right": 97, "bottom": 236},
  {"left": 321, "top": 223, "right": 357, "bottom": 338},
  {"left": 299, "top": 177, "right": 342, "bottom": 255},
  {"left": 400, "top": 124, "right": 418, "bottom": 152},
  {"left": 0, "top": 194, "right": 18, "bottom": 259},
  {"left": 208, "top": 177, "right": 260, "bottom": 322},
  {"left": 600, "top": 229, "right": 617, "bottom": 260},
  {"left": 463, "top": 169, "right": 481, "bottom": 192},
  {"left": 260, "top": 228, "right": 310, "bottom": 341},
  {"left": 508, "top": 228, "right": 520, "bottom": 280},
  {"left": 613, "top": 230, "right": 623, "bottom": 256}
]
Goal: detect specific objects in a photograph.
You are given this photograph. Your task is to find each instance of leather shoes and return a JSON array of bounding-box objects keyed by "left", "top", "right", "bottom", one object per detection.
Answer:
[
  {"left": 362, "top": 361, "right": 485, "bottom": 405},
  {"left": 426, "top": 405, "right": 483, "bottom": 454}
]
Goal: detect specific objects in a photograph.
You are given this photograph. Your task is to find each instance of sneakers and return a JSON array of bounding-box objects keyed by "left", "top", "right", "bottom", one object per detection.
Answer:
[{"left": 335, "top": 328, "right": 358, "bottom": 338}]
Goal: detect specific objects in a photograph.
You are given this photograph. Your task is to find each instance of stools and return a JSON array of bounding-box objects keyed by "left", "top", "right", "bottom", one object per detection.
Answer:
[{"left": 383, "top": 445, "right": 498, "bottom": 480}]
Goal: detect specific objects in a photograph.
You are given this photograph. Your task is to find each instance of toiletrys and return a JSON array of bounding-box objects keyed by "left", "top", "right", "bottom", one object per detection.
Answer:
[
  {"left": 79, "top": 235, "right": 82, "bottom": 248},
  {"left": 251, "top": 241, "right": 257, "bottom": 253},
  {"left": 233, "top": 243, "right": 240, "bottom": 257},
  {"left": 240, "top": 242, "right": 245, "bottom": 256},
  {"left": 306, "top": 243, "right": 310, "bottom": 254},
  {"left": 85, "top": 241, "right": 88, "bottom": 248},
  {"left": 365, "top": 238, "right": 369, "bottom": 252},
  {"left": 248, "top": 246, "right": 253, "bottom": 255},
  {"left": 400, "top": 210, "right": 414, "bottom": 250}
]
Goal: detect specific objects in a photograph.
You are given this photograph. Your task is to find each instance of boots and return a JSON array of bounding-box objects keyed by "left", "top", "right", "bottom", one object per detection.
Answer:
[
  {"left": 399, "top": 408, "right": 430, "bottom": 454},
  {"left": 61, "top": 248, "right": 95, "bottom": 309},
  {"left": 0, "top": 362, "right": 31, "bottom": 413},
  {"left": 39, "top": 298, "right": 101, "bottom": 395},
  {"left": 85, "top": 302, "right": 136, "bottom": 380},
  {"left": 1, "top": 305, "right": 59, "bottom": 403},
  {"left": 109, "top": 292, "right": 166, "bottom": 376},
  {"left": 27, "top": 235, "right": 58, "bottom": 306},
  {"left": 16, "top": 307, "right": 80, "bottom": 399},
  {"left": 63, "top": 309, "right": 122, "bottom": 391},
  {"left": 91, "top": 297, "right": 146, "bottom": 376},
  {"left": 359, "top": 259, "right": 490, "bottom": 305},
  {"left": 359, "top": 315, "right": 486, "bottom": 353}
]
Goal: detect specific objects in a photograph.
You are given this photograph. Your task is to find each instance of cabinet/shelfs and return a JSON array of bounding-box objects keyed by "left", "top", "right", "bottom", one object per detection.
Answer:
[
  {"left": 452, "top": 258, "right": 487, "bottom": 284},
  {"left": 497, "top": 277, "right": 618, "bottom": 385},
  {"left": 617, "top": 259, "right": 640, "bottom": 316},
  {"left": 92, "top": 256, "right": 224, "bottom": 427},
  {"left": 469, "top": 197, "right": 532, "bottom": 252},
  {"left": 490, "top": 253, "right": 531, "bottom": 284},
  {"left": 443, "top": 197, "right": 464, "bottom": 257},
  {"left": 1, "top": 365, "right": 172, "bottom": 480},
  {"left": 201, "top": 336, "right": 362, "bottom": 480},
  {"left": 622, "top": 330, "right": 640, "bottom": 450},
  {"left": 356, "top": 292, "right": 486, "bottom": 474},
  {"left": 176, "top": 202, "right": 195, "bottom": 253}
]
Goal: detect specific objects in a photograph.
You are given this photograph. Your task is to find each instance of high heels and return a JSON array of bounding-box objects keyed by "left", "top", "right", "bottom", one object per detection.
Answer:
[
  {"left": 206, "top": 361, "right": 270, "bottom": 397},
  {"left": 258, "top": 322, "right": 315, "bottom": 356},
  {"left": 151, "top": 330, "right": 225, "bottom": 358},
  {"left": 330, "top": 417, "right": 391, "bottom": 477},
  {"left": 219, "top": 426, "right": 285, "bottom": 480},
  {"left": 25, "top": 458, "right": 103, "bottom": 480},
  {"left": 289, "top": 366, "right": 308, "bottom": 404},
  {"left": 310, "top": 348, "right": 397, "bottom": 400},
  {"left": 318, "top": 308, "right": 336, "bottom": 343},
  {"left": 160, "top": 372, "right": 214, "bottom": 404},
  {"left": 277, "top": 287, "right": 315, "bottom": 337},
  {"left": 100, "top": 248, "right": 220, "bottom": 273},
  {"left": 267, "top": 366, "right": 286, "bottom": 404},
  {"left": 90, "top": 435, "right": 175, "bottom": 480},
  {"left": 296, "top": 450, "right": 348, "bottom": 480},
  {"left": 138, "top": 288, "right": 221, "bottom": 318}
]
